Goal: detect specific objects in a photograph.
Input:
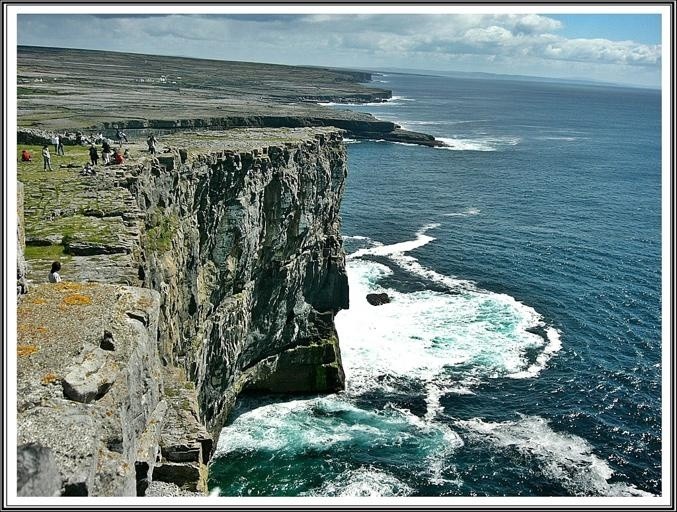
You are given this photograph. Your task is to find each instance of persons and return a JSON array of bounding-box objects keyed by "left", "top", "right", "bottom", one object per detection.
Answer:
[
  {"left": 22, "top": 130, "right": 160, "bottom": 171},
  {"left": 48, "top": 262, "right": 62, "bottom": 283}
]
